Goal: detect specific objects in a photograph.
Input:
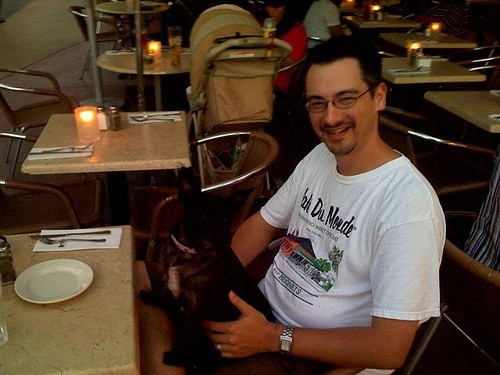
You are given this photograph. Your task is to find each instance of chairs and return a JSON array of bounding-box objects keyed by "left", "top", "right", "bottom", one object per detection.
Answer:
[{"left": 0, "top": 0, "right": 500, "bottom": 375}]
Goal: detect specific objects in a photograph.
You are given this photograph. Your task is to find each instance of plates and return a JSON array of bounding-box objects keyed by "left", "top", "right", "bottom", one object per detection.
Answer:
[{"left": 14, "top": 258, "right": 94, "bottom": 304}]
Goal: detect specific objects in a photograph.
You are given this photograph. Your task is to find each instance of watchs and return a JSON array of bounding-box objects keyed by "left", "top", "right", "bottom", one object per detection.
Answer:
[{"left": 279, "top": 326, "right": 294, "bottom": 355}]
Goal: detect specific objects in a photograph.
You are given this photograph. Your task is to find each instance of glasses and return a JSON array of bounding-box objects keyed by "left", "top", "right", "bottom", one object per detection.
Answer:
[{"left": 306, "top": 81, "right": 379, "bottom": 113}]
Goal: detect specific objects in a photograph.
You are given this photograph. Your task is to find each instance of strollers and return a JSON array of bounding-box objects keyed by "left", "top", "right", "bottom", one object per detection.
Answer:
[{"left": 184, "top": 4, "right": 294, "bottom": 211}]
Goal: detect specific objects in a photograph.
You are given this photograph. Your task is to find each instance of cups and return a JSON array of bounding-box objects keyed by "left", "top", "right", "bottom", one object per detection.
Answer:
[
  {"left": 264, "top": 16, "right": 277, "bottom": 31},
  {"left": 168, "top": 25, "right": 182, "bottom": 50}
]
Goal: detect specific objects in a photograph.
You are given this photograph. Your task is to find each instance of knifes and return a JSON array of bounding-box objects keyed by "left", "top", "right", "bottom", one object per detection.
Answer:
[
  {"left": 30, "top": 230, "right": 111, "bottom": 238},
  {"left": 130, "top": 113, "right": 180, "bottom": 118},
  {"left": 30, "top": 151, "right": 94, "bottom": 155}
]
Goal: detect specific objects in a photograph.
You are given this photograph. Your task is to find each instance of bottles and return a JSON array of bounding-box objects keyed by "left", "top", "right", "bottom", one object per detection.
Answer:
[
  {"left": 109, "top": 107, "right": 121, "bottom": 131},
  {"left": 0, "top": 235, "right": 16, "bottom": 286},
  {"left": 410, "top": 48, "right": 423, "bottom": 66},
  {"left": 96, "top": 105, "right": 108, "bottom": 130}
]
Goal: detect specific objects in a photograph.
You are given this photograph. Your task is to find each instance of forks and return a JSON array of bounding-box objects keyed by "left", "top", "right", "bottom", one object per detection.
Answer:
[
  {"left": 395, "top": 66, "right": 423, "bottom": 73},
  {"left": 42, "top": 143, "right": 94, "bottom": 152},
  {"left": 129, "top": 116, "right": 174, "bottom": 122},
  {"left": 31, "top": 234, "right": 107, "bottom": 245}
]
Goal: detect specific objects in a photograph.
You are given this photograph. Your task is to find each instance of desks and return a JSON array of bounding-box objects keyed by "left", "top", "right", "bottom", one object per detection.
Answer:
[
  {"left": 97, "top": 45, "right": 191, "bottom": 112},
  {"left": 23, "top": 112, "right": 191, "bottom": 227},
  {"left": 424, "top": 89, "right": 500, "bottom": 156},
  {"left": 379, "top": 32, "right": 477, "bottom": 49},
  {"left": 382, "top": 57, "right": 487, "bottom": 83},
  {"left": 80, "top": 1, "right": 168, "bottom": 80},
  {"left": 0, "top": 224, "right": 140, "bottom": 375},
  {"left": 343, "top": 15, "right": 421, "bottom": 28}
]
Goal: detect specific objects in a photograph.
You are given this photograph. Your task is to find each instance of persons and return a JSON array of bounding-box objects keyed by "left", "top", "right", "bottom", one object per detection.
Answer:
[
  {"left": 303, "top": 0, "right": 343, "bottom": 47},
  {"left": 465, "top": 154, "right": 500, "bottom": 272},
  {"left": 203, "top": 38, "right": 446, "bottom": 375},
  {"left": 260, "top": 0, "right": 307, "bottom": 95}
]
